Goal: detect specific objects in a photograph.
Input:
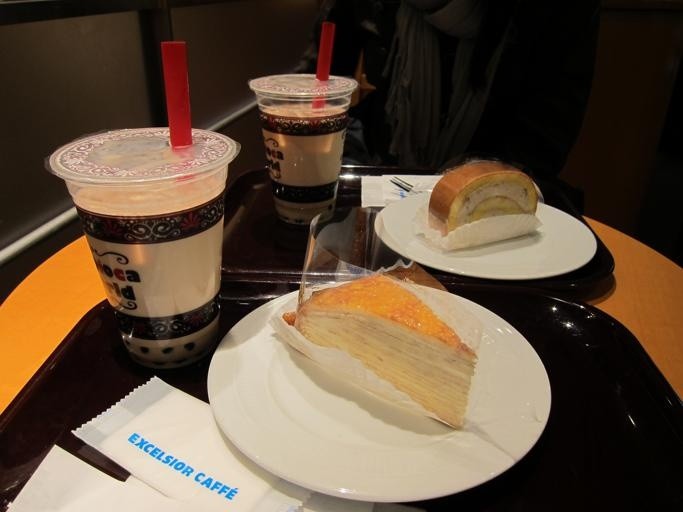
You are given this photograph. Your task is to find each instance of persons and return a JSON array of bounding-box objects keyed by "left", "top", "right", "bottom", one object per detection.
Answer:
[{"left": 294, "top": 0, "right": 602, "bottom": 214}]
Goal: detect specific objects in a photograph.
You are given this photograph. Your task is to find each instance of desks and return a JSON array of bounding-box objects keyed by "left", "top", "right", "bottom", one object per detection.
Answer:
[{"left": 0, "top": 167, "right": 682, "bottom": 509}]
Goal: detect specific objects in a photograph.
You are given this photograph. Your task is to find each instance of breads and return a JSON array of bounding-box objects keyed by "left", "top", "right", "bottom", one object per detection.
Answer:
[
  {"left": 298, "top": 273, "right": 479, "bottom": 428},
  {"left": 428, "top": 160, "right": 540, "bottom": 238}
]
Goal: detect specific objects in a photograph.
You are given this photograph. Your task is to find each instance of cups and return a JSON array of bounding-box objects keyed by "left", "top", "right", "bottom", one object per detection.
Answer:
[
  {"left": 50, "top": 126, "right": 237, "bottom": 370},
  {"left": 247, "top": 74, "right": 359, "bottom": 225}
]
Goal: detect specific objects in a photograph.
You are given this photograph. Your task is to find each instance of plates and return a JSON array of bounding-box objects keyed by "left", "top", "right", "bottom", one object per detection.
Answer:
[
  {"left": 206, "top": 279, "right": 553, "bottom": 503},
  {"left": 374, "top": 191, "right": 597, "bottom": 279}
]
